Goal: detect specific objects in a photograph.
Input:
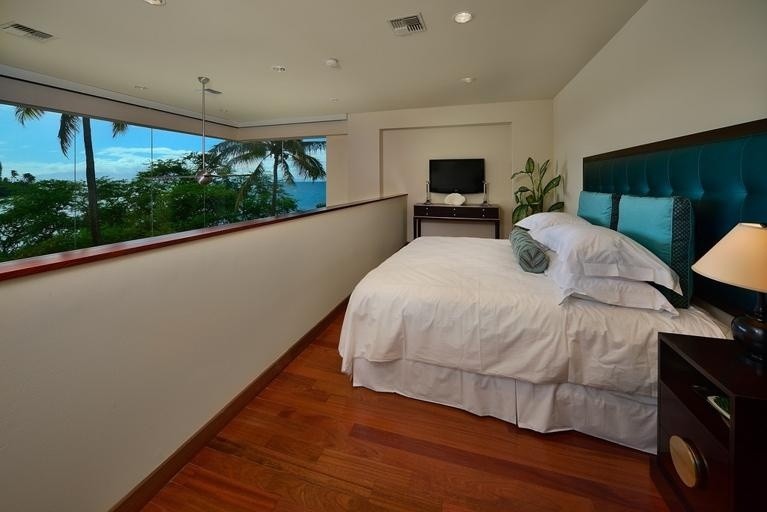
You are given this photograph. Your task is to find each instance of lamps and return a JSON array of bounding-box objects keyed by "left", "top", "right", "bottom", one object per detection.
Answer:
[{"left": 691, "top": 223, "right": 767, "bottom": 371}]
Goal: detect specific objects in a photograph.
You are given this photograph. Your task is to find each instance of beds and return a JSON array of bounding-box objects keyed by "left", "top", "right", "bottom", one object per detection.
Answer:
[{"left": 338, "top": 118, "right": 767, "bottom": 455}]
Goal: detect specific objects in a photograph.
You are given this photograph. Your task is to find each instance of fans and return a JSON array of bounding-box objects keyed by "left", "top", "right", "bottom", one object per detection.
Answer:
[{"left": 147, "top": 77, "right": 252, "bottom": 184}]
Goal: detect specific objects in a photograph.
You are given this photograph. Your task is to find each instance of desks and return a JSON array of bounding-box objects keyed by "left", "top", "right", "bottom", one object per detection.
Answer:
[{"left": 413, "top": 203, "right": 500, "bottom": 239}]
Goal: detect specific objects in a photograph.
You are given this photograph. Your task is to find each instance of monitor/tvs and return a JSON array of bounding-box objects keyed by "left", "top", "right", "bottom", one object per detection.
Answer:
[{"left": 429, "top": 158, "right": 485, "bottom": 195}]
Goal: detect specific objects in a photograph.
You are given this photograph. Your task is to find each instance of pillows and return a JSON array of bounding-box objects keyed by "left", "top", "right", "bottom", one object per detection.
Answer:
[
  {"left": 617, "top": 194, "right": 695, "bottom": 308},
  {"left": 543, "top": 249, "right": 679, "bottom": 318},
  {"left": 577, "top": 191, "right": 620, "bottom": 231},
  {"left": 531, "top": 223, "right": 683, "bottom": 298},
  {"left": 509, "top": 227, "right": 548, "bottom": 273},
  {"left": 515, "top": 212, "right": 592, "bottom": 234}
]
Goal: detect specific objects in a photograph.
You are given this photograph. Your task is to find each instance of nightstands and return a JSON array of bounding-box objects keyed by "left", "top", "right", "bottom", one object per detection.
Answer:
[{"left": 658, "top": 332, "right": 767, "bottom": 512}]
